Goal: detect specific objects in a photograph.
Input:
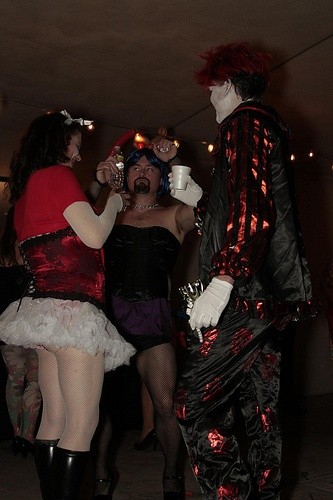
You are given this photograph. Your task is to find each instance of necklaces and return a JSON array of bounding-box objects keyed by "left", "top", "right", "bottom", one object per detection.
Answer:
[{"left": 127, "top": 202, "right": 160, "bottom": 209}]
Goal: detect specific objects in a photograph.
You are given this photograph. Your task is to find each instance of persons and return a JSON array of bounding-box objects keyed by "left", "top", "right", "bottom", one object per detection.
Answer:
[
  {"left": 0, "top": 204, "right": 42, "bottom": 459},
  {"left": 86, "top": 140, "right": 196, "bottom": 500},
  {"left": 172, "top": 42, "right": 312, "bottom": 500},
  {"left": 0, "top": 104, "right": 138, "bottom": 500}
]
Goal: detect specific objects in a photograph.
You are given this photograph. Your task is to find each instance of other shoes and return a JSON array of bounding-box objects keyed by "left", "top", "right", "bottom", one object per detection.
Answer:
[
  {"left": 93, "top": 470, "right": 115, "bottom": 500},
  {"left": 163, "top": 464, "right": 185, "bottom": 500}
]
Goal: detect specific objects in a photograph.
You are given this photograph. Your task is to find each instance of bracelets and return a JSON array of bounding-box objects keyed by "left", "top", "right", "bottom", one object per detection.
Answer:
[
  {"left": 94, "top": 177, "right": 106, "bottom": 188},
  {"left": 166, "top": 154, "right": 178, "bottom": 165}
]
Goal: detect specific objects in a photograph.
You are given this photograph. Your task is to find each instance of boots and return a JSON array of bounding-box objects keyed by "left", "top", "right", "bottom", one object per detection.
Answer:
[
  {"left": 33, "top": 439, "right": 60, "bottom": 500},
  {"left": 52, "top": 444, "right": 90, "bottom": 500}
]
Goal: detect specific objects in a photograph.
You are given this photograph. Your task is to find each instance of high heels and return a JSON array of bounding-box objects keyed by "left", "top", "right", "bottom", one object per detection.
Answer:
[
  {"left": 135, "top": 429, "right": 158, "bottom": 451},
  {"left": 21, "top": 442, "right": 39, "bottom": 458},
  {"left": 11, "top": 436, "right": 22, "bottom": 456}
]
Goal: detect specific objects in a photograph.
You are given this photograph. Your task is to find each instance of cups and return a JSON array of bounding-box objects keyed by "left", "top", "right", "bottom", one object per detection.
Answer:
[{"left": 170, "top": 165, "right": 192, "bottom": 191}]
